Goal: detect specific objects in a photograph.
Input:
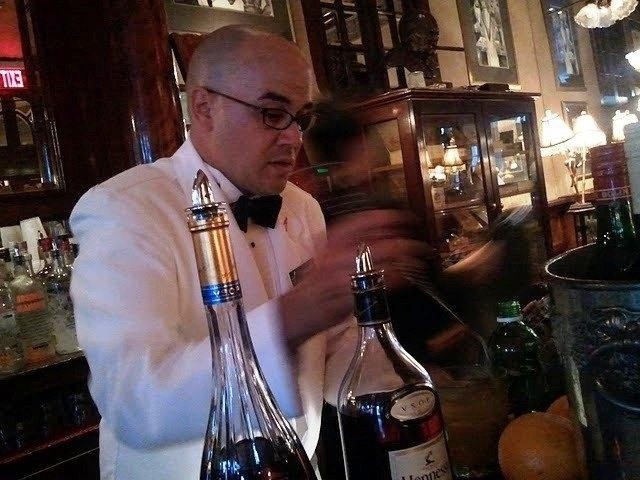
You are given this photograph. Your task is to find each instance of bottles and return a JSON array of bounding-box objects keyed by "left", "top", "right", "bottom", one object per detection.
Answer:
[
  {"left": 184, "top": 203, "right": 316, "bottom": 480},
  {"left": 0, "top": 235, "right": 80, "bottom": 374},
  {"left": 588, "top": 143, "right": 640, "bottom": 282},
  {"left": 336, "top": 239, "right": 454, "bottom": 480},
  {"left": 491, "top": 300, "right": 547, "bottom": 423}
]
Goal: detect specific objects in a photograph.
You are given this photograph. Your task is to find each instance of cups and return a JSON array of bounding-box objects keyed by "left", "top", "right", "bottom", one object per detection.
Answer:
[
  {"left": 19, "top": 216, "right": 52, "bottom": 276},
  {"left": 433, "top": 364, "right": 509, "bottom": 478}
]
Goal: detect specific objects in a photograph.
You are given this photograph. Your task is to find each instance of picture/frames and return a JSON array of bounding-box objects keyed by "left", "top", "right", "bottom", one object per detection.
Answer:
[{"left": 540, "top": 1, "right": 587, "bottom": 92}]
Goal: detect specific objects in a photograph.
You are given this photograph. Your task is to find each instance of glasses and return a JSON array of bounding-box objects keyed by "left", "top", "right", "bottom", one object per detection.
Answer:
[{"left": 199, "top": 85, "right": 324, "bottom": 135}]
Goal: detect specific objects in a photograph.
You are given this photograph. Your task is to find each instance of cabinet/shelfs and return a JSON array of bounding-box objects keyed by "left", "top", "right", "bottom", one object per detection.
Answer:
[{"left": 326, "top": 87, "right": 553, "bottom": 271}]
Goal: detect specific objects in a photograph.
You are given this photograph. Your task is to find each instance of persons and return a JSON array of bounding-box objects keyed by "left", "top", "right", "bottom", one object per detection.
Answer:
[{"left": 69, "top": 25, "right": 441, "bottom": 479}]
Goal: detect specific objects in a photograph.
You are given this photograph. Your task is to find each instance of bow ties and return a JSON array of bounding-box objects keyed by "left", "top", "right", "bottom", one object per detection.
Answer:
[{"left": 234, "top": 191, "right": 282, "bottom": 234}]
